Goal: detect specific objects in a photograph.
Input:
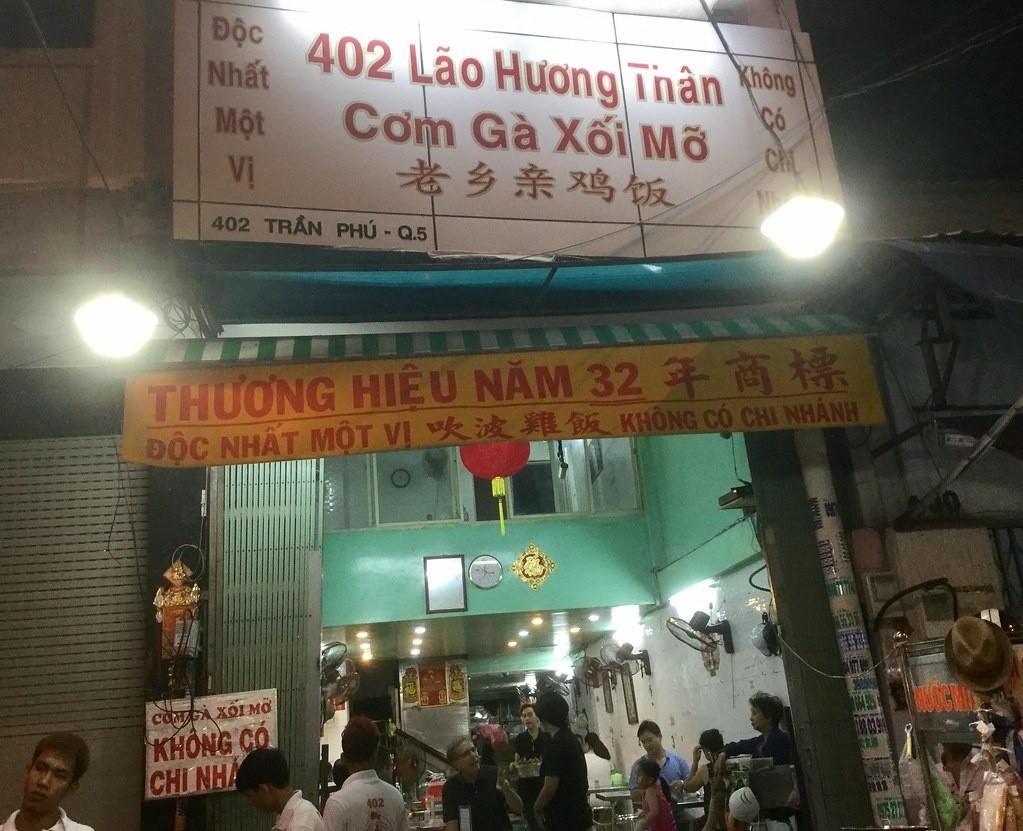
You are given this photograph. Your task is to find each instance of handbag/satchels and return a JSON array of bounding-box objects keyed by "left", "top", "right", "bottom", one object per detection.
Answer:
[{"left": 898, "top": 729, "right": 958, "bottom": 831}]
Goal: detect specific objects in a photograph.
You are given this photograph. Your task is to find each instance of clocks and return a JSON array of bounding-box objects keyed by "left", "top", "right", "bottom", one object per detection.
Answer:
[
  {"left": 468, "top": 555, "right": 504, "bottom": 590},
  {"left": 391, "top": 469, "right": 411, "bottom": 487}
]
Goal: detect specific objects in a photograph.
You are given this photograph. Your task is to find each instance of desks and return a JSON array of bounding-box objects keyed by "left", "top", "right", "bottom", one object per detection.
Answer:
[
  {"left": 633, "top": 797, "right": 706, "bottom": 830},
  {"left": 596, "top": 791, "right": 633, "bottom": 831},
  {"left": 587, "top": 785, "right": 630, "bottom": 831}
]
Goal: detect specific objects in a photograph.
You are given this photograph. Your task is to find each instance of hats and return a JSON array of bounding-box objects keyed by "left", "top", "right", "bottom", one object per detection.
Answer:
[{"left": 944, "top": 617, "right": 1013, "bottom": 692}]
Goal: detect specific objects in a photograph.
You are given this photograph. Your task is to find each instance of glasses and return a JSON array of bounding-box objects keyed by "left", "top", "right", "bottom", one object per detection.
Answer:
[{"left": 454, "top": 746, "right": 478, "bottom": 762}]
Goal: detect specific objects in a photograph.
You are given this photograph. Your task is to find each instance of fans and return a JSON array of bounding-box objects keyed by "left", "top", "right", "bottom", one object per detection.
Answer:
[
  {"left": 545, "top": 672, "right": 581, "bottom": 696},
  {"left": 396, "top": 743, "right": 427, "bottom": 818},
  {"left": 510, "top": 687, "right": 545, "bottom": 703},
  {"left": 601, "top": 642, "right": 652, "bottom": 676},
  {"left": 419, "top": 446, "right": 451, "bottom": 481},
  {"left": 664, "top": 611, "right": 732, "bottom": 653},
  {"left": 319, "top": 641, "right": 360, "bottom": 704},
  {"left": 575, "top": 655, "right": 617, "bottom": 686}
]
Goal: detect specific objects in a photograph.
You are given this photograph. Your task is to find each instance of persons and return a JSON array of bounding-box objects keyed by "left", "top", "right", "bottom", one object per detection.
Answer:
[
  {"left": 629, "top": 721, "right": 691, "bottom": 817},
  {"left": 236, "top": 749, "right": 328, "bottom": 831},
  {"left": 576, "top": 733, "right": 618, "bottom": 806},
  {"left": 685, "top": 729, "right": 723, "bottom": 831},
  {"left": 633, "top": 759, "right": 677, "bottom": 831},
  {"left": 323, "top": 716, "right": 410, "bottom": 831},
  {"left": 442, "top": 738, "right": 524, "bottom": 831},
  {"left": 514, "top": 692, "right": 592, "bottom": 831},
  {"left": 0, "top": 732, "right": 94, "bottom": 831},
  {"left": 714, "top": 694, "right": 800, "bottom": 831}
]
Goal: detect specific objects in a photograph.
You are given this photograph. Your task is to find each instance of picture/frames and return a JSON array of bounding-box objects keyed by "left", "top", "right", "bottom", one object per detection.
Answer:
[
  {"left": 423, "top": 554, "right": 468, "bottom": 614},
  {"left": 602, "top": 670, "right": 614, "bottom": 712},
  {"left": 620, "top": 662, "right": 639, "bottom": 723}
]
